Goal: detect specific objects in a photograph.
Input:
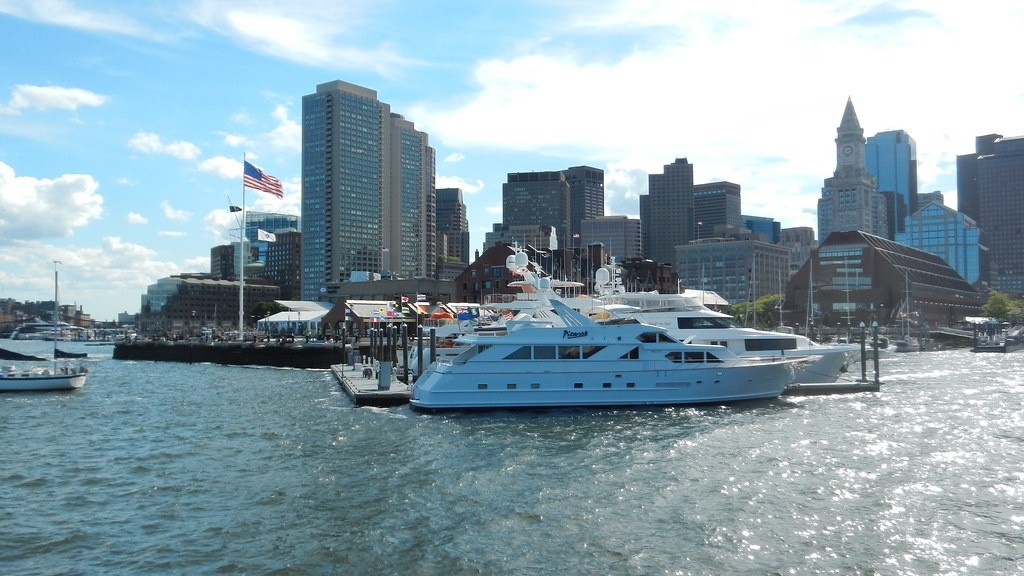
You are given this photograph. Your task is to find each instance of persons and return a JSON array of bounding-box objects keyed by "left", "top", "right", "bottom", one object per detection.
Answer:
[
  {"left": 448, "top": 340, "right": 453, "bottom": 348},
  {"left": 127, "top": 327, "right": 358, "bottom": 343},
  {"left": 435, "top": 338, "right": 441, "bottom": 348}
]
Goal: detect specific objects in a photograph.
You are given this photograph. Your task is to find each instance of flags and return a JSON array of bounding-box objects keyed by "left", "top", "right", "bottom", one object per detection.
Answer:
[
  {"left": 345, "top": 291, "right": 514, "bottom": 322},
  {"left": 244, "top": 160, "right": 284, "bottom": 198},
  {"left": 573, "top": 233, "right": 579, "bottom": 237}
]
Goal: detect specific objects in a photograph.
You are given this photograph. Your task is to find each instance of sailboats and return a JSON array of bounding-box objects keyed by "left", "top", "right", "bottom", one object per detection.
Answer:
[
  {"left": 408, "top": 228, "right": 935, "bottom": 410},
  {"left": 0, "top": 257, "right": 88, "bottom": 391}
]
